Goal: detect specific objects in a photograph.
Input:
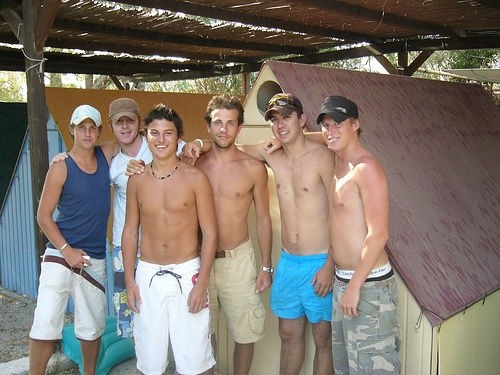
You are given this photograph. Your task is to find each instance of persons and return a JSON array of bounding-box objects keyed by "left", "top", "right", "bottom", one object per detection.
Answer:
[
  {"left": 124, "top": 94, "right": 273, "bottom": 375},
  {"left": 49, "top": 97, "right": 200, "bottom": 375},
  {"left": 121, "top": 104, "right": 218, "bottom": 375},
  {"left": 29, "top": 104, "right": 145, "bottom": 375},
  {"left": 265, "top": 95, "right": 403, "bottom": 375},
  {"left": 182, "top": 93, "right": 339, "bottom": 375}
]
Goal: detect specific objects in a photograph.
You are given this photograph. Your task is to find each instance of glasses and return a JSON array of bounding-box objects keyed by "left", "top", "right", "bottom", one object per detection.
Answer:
[{"left": 265, "top": 98, "right": 300, "bottom": 112}]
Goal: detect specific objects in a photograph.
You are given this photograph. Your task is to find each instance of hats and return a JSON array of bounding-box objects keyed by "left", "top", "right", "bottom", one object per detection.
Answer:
[
  {"left": 70, "top": 104, "right": 102, "bottom": 128},
  {"left": 263, "top": 93, "right": 304, "bottom": 122},
  {"left": 108, "top": 98, "right": 140, "bottom": 125},
  {"left": 316, "top": 95, "right": 358, "bottom": 126}
]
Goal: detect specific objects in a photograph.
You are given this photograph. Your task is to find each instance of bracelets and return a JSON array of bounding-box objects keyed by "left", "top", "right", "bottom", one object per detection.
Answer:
[
  {"left": 195, "top": 138, "right": 203, "bottom": 148},
  {"left": 59, "top": 243, "right": 68, "bottom": 253}
]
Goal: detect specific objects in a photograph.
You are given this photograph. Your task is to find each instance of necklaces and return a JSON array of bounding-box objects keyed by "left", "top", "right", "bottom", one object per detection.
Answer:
[{"left": 151, "top": 157, "right": 181, "bottom": 179}]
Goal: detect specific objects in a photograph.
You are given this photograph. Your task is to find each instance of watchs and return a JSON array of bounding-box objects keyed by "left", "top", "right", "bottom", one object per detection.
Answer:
[{"left": 260, "top": 266, "right": 274, "bottom": 273}]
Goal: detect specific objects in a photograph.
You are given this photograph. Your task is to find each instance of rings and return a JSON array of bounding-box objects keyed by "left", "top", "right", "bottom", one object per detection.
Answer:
[{"left": 206, "top": 302, "right": 208, "bottom": 305}]
